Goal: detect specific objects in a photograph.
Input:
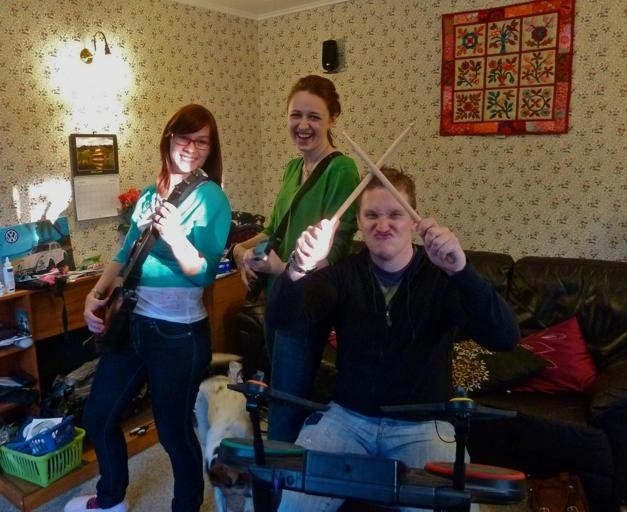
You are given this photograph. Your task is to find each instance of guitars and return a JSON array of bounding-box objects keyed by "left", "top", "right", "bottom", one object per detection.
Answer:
[
  {"left": 244, "top": 238, "right": 281, "bottom": 303},
  {"left": 85, "top": 170, "right": 207, "bottom": 345}
]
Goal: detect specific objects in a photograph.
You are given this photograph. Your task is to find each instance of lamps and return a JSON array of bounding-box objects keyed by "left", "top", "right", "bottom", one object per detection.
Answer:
[{"left": 80, "top": 29, "right": 116, "bottom": 67}]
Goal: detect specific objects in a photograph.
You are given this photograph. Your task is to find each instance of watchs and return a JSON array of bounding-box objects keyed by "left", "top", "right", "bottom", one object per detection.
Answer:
[{"left": 290, "top": 251, "right": 318, "bottom": 276}]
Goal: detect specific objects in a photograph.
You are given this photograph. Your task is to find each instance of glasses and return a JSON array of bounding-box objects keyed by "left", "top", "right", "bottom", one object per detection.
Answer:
[{"left": 170, "top": 134, "right": 211, "bottom": 150}]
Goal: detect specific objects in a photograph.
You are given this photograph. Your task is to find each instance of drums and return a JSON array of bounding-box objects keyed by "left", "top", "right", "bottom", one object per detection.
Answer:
[
  {"left": 427, "top": 460, "right": 529, "bottom": 507},
  {"left": 210, "top": 439, "right": 306, "bottom": 489}
]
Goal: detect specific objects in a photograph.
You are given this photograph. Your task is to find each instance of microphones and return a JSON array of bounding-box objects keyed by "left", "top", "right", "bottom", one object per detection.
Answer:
[
  {"left": 448, "top": 387, "right": 475, "bottom": 438},
  {"left": 246, "top": 370, "right": 267, "bottom": 411}
]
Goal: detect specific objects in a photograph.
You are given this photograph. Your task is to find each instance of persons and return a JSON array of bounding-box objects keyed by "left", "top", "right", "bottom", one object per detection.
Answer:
[
  {"left": 262, "top": 167, "right": 523, "bottom": 510},
  {"left": 228, "top": 75, "right": 361, "bottom": 442},
  {"left": 66, "top": 104, "right": 231, "bottom": 512}
]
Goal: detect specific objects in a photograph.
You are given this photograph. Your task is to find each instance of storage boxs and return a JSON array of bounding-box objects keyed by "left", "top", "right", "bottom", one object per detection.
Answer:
[{"left": 1, "top": 415, "right": 84, "bottom": 488}]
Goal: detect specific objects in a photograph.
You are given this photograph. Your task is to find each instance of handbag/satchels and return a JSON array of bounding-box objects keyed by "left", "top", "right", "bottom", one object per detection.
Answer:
[{"left": 527, "top": 473, "right": 589, "bottom": 512}]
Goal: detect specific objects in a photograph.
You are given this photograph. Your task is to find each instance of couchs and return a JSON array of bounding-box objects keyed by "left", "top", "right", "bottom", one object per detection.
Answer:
[{"left": 232, "top": 243, "right": 626, "bottom": 476}]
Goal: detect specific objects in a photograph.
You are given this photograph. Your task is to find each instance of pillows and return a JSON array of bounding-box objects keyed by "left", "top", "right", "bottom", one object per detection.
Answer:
[{"left": 519, "top": 317, "right": 599, "bottom": 393}]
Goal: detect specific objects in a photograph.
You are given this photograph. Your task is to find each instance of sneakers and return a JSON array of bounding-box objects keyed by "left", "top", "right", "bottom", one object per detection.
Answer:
[{"left": 65, "top": 496, "right": 129, "bottom": 512}]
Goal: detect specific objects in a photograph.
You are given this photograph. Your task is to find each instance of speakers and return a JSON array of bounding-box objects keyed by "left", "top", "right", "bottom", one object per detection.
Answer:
[{"left": 322, "top": 40, "right": 338, "bottom": 73}]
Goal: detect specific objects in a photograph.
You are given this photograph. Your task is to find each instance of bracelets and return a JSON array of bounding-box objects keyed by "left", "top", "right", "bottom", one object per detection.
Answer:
[{"left": 90, "top": 287, "right": 106, "bottom": 297}]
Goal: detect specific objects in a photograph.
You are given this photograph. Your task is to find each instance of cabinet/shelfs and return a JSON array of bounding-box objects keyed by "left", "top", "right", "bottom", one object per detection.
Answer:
[{"left": 0, "top": 266, "right": 266, "bottom": 512}]
